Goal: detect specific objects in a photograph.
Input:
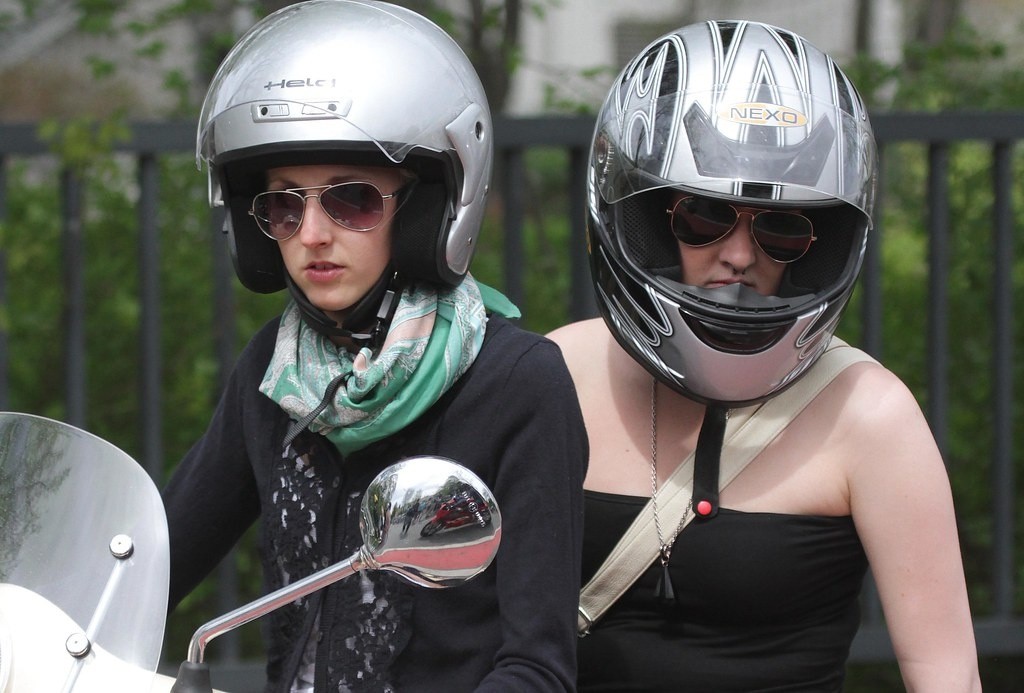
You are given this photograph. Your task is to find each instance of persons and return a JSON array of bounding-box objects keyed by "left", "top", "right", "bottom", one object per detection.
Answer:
[
  {"left": 161, "top": 0, "right": 591, "bottom": 693},
  {"left": 541, "top": 18, "right": 986, "bottom": 693},
  {"left": 402, "top": 504, "right": 415, "bottom": 534},
  {"left": 373, "top": 497, "right": 380, "bottom": 543},
  {"left": 378, "top": 501, "right": 392, "bottom": 551},
  {"left": 410, "top": 499, "right": 420, "bottom": 526}
]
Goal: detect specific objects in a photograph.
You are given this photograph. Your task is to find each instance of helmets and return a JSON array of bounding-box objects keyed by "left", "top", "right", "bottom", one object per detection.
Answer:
[
  {"left": 586, "top": 20, "right": 879, "bottom": 406},
  {"left": 195, "top": 0, "right": 493, "bottom": 292}
]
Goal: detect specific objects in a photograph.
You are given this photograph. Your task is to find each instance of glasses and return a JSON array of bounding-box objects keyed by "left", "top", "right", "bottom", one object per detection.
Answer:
[
  {"left": 248, "top": 180, "right": 410, "bottom": 240},
  {"left": 666, "top": 196, "right": 817, "bottom": 263}
]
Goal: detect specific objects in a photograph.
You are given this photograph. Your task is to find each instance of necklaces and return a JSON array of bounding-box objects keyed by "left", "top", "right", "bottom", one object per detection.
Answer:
[{"left": 638, "top": 368, "right": 715, "bottom": 602}]
